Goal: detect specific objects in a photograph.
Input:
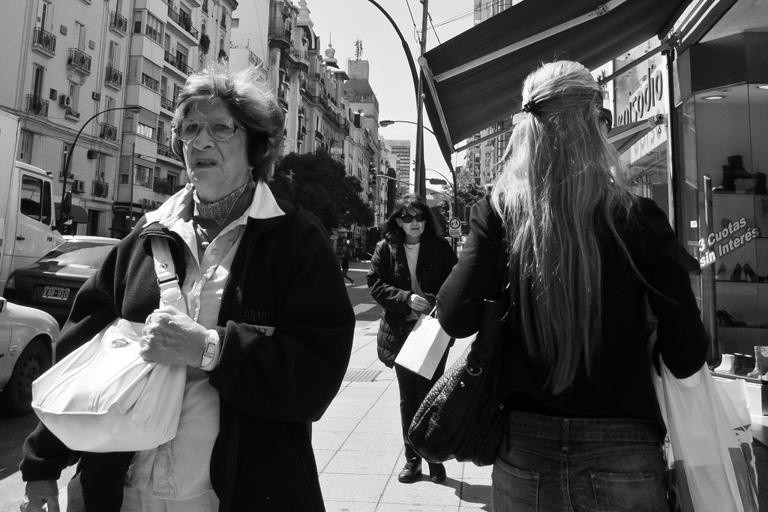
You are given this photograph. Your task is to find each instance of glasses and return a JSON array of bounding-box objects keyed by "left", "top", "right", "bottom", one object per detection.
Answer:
[
  {"left": 400, "top": 213, "right": 427, "bottom": 223},
  {"left": 596, "top": 108, "right": 613, "bottom": 132},
  {"left": 176, "top": 115, "right": 249, "bottom": 142}
]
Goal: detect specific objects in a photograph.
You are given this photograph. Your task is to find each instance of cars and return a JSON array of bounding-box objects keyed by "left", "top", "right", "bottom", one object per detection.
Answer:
[
  {"left": 0, "top": 294, "right": 61, "bottom": 413},
  {"left": 6, "top": 241, "right": 121, "bottom": 326}
]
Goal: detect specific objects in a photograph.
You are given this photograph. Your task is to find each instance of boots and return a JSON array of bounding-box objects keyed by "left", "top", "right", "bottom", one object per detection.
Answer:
[
  {"left": 713, "top": 156, "right": 768, "bottom": 195},
  {"left": 714, "top": 346, "right": 768, "bottom": 381}
]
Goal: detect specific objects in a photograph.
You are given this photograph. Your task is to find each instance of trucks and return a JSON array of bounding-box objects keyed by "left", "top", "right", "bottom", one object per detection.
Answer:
[{"left": 0, "top": 106, "right": 68, "bottom": 295}]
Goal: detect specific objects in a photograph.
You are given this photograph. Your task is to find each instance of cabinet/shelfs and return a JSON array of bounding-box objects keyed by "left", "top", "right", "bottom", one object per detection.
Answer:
[{"left": 702, "top": 173, "right": 766, "bottom": 370}]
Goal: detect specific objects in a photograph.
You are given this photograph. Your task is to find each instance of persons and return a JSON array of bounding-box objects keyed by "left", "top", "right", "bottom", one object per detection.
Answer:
[
  {"left": 341, "top": 249, "right": 355, "bottom": 286},
  {"left": 434, "top": 61, "right": 710, "bottom": 511},
  {"left": 17, "top": 58, "right": 359, "bottom": 512},
  {"left": 364, "top": 193, "right": 448, "bottom": 485}
]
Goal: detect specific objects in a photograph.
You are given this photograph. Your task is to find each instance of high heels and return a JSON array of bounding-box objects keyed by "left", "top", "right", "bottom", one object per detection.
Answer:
[
  {"left": 743, "top": 263, "right": 765, "bottom": 282},
  {"left": 718, "top": 309, "right": 752, "bottom": 327},
  {"left": 730, "top": 263, "right": 742, "bottom": 281}
]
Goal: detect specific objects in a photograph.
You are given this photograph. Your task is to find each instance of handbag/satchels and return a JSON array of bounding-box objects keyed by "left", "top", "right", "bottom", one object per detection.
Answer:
[
  {"left": 394, "top": 305, "right": 452, "bottom": 381},
  {"left": 32, "top": 318, "right": 189, "bottom": 454},
  {"left": 407, "top": 321, "right": 507, "bottom": 466}
]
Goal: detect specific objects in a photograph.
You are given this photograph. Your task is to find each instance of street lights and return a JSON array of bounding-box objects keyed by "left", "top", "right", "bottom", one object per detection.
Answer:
[
  {"left": 380, "top": 119, "right": 458, "bottom": 257},
  {"left": 58, "top": 105, "right": 144, "bottom": 236},
  {"left": 411, "top": 166, "right": 455, "bottom": 195}
]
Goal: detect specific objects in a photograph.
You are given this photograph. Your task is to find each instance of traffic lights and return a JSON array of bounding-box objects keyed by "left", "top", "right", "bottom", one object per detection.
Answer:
[{"left": 370, "top": 167, "right": 377, "bottom": 186}]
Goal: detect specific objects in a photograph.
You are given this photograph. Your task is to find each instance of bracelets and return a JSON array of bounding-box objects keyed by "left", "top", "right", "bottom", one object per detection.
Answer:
[{"left": 199, "top": 329, "right": 219, "bottom": 371}]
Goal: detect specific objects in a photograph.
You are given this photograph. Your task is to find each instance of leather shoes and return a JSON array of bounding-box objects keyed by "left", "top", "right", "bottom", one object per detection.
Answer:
[
  {"left": 399, "top": 458, "right": 422, "bottom": 481},
  {"left": 715, "top": 264, "right": 727, "bottom": 280},
  {"left": 429, "top": 462, "right": 445, "bottom": 482}
]
